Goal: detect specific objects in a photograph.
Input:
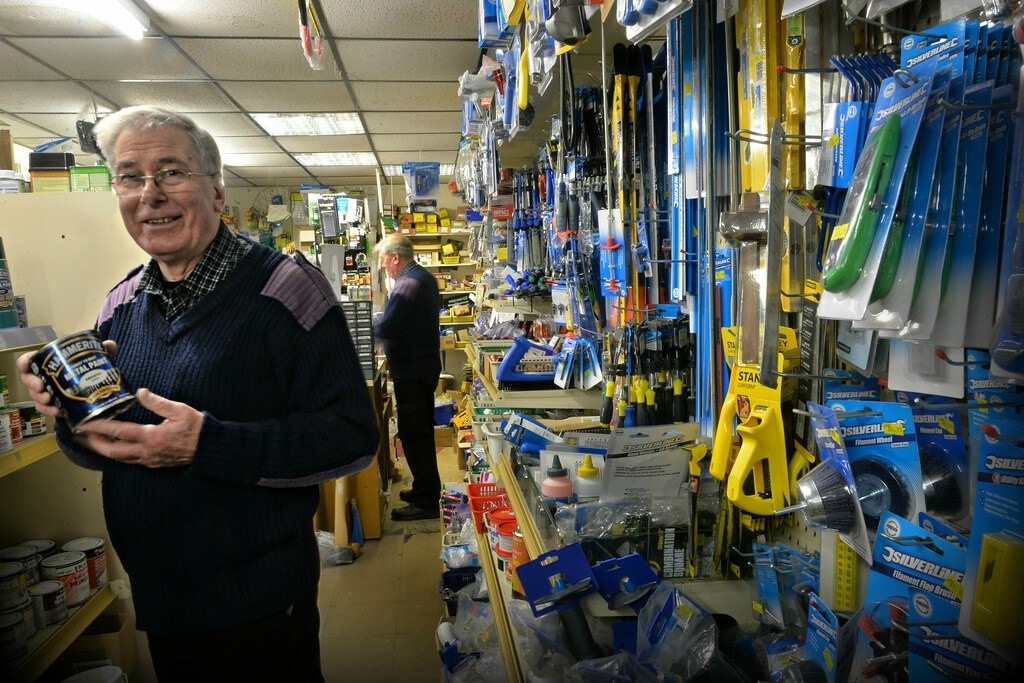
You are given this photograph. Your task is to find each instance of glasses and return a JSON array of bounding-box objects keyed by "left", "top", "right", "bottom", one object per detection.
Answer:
[{"left": 108, "top": 169, "right": 215, "bottom": 198}]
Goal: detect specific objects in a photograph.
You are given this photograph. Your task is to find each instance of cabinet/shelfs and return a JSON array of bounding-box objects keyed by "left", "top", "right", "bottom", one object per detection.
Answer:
[
  {"left": 1, "top": 325, "right": 122, "bottom": 683},
  {"left": 374, "top": 226, "right": 481, "bottom": 354},
  {"left": 458, "top": 336, "right": 661, "bottom": 683},
  {"left": 313, "top": 221, "right": 369, "bottom": 294},
  {"left": 308, "top": 354, "right": 398, "bottom": 543}
]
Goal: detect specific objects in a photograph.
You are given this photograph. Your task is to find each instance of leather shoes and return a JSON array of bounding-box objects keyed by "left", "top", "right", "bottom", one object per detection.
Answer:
[
  {"left": 399, "top": 490, "right": 418, "bottom": 503},
  {"left": 390, "top": 504, "right": 440, "bottom": 521}
]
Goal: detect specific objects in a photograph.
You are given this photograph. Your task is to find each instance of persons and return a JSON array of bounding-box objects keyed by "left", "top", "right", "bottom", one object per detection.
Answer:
[
  {"left": 11, "top": 105, "right": 384, "bottom": 682},
  {"left": 370, "top": 234, "right": 447, "bottom": 521}
]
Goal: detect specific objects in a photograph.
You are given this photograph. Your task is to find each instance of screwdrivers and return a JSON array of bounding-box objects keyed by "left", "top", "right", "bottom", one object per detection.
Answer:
[
  {"left": 554, "top": 125, "right": 568, "bottom": 231},
  {"left": 568, "top": 179, "right": 580, "bottom": 234},
  {"left": 599, "top": 316, "right": 693, "bottom": 428}
]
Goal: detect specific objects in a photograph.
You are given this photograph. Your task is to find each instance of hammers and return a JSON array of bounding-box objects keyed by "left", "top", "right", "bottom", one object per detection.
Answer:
[{"left": 718, "top": 189, "right": 770, "bottom": 368}]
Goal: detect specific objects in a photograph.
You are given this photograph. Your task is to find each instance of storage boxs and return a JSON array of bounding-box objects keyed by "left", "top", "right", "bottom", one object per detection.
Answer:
[
  {"left": 438, "top": 327, "right": 470, "bottom": 352},
  {"left": 411, "top": 241, "right": 474, "bottom": 265},
  {"left": 433, "top": 398, "right": 456, "bottom": 426},
  {"left": 67, "top": 164, "right": 114, "bottom": 193},
  {"left": 466, "top": 482, "right": 511, "bottom": 514},
  {"left": 341, "top": 302, "right": 374, "bottom": 381},
  {"left": 468, "top": 497, "right": 511, "bottom": 535},
  {"left": 443, "top": 543, "right": 480, "bottom": 570},
  {"left": 63, "top": 612, "right": 140, "bottom": 677},
  {"left": 429, "top": 272, "right": 481, "bottom": 293},
  {"left": 458, "top": 394, "right": 470, "bottom": 409},
  {"left": 441, "top": 532, "right": 467, "bottom": 550},
  {"left": 460, "top": 381, "right": 473, "bottom": 395},
  {"left": 27, "top": 153, "right": 77, "bottom": 171},
  {"left": 434, "top": 378, "right": 455, "bottom": 397},
  {"left": 452, "top": 410, "right": 473, "bottom": 429},
  {"left": 438, "top": 565, "right": 492, "bottom": 618},
  {"left": 434, "top": 617, "right": 484, "bottom": 675},
  {"left": 453, "top": 428, "right": 475, "bottom": 471},
  {"left": 377, "top": 202, "right": 472, "bottom": 232},
  {"left": 446, "top": 389, "right": 464, "bottom": 407},
  {"left": 438, "top": 297, "right": 477, "bottom": 323},
  {"left": 434, "top": 426, "right": 456, "bottom": 448},
  {"left": 30, "top": 170, "right": 73, "bottom": 193}
]
